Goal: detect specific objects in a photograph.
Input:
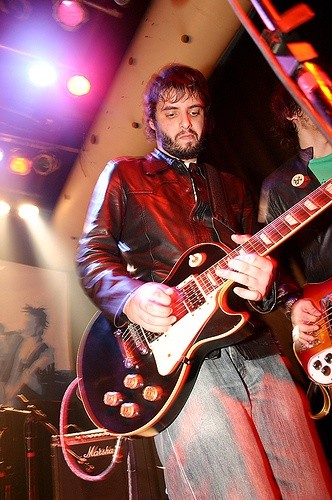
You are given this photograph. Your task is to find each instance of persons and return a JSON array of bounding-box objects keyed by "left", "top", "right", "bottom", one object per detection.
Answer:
[
  {"left": 75, "top": 64, "right": 332, "bottom": 499},
  {"left": 1, "top": 305, "right": 56, "bottom": 408},
  {"left": 256, "top": 81, "right": 332, "bottom": 471}
]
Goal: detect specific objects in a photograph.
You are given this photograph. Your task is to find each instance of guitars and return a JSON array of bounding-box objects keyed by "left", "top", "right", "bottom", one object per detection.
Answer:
[
  {"left": 291, "top": 277, "right": 332, "bottom": 388},
  {"left": 75, "top": 176, "right": 332, "bottom": 438}
]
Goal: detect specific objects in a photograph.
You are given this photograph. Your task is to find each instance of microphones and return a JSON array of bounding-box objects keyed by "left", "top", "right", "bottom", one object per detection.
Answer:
[{"left": 85, "top": 465, "right": 94, "bottom": 473}]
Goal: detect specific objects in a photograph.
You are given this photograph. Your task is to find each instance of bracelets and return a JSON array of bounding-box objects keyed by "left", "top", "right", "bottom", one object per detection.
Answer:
[{"left": 283, "top": 294, "right": 301, "bottom": 321}]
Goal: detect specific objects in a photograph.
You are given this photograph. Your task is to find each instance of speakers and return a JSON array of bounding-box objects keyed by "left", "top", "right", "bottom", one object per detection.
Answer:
[{"left": 50, "top": 429, "right": 169, "bottom": 500}]
